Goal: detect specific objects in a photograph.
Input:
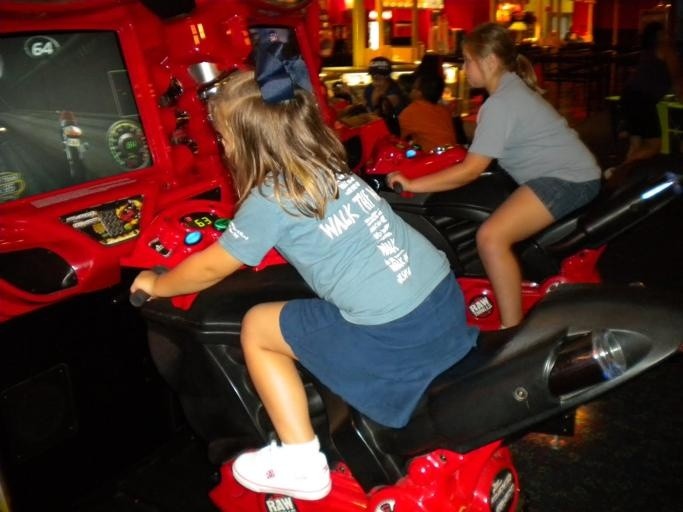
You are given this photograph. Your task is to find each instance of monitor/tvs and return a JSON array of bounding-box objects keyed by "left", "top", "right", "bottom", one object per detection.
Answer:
[
  {"left": 248, "top": 25, "right": 292, "bottom": 45},
  {"left": 0, "top": 27, "right": 156, "bottom": 205}
]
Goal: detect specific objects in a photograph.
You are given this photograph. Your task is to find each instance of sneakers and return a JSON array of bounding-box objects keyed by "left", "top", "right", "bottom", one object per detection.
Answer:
[{"left": 232, "top": 442, "right": 332, "bottom": 501}]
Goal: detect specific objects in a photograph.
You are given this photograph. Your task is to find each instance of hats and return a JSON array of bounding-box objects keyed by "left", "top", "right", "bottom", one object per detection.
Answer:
[{"left": 368, "top": 57, "right": 392, "bottom": 74}]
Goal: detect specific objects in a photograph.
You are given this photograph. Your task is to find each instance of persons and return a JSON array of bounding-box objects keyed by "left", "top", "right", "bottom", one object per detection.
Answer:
[
  {"left": 131, "top": 65, "right": 479, "bottom": 501},
  {"left": 386, "top": 23, "right": 603, "bottom": 329},
  {"left": 620, "top": 20, "right": 683, "bottom": 163},
  {"left": 563, "top": 31, "right": 584, "bottom": 44},
  {"left": 363, "top": 53, "right": 456, "bottom": 151}
]
